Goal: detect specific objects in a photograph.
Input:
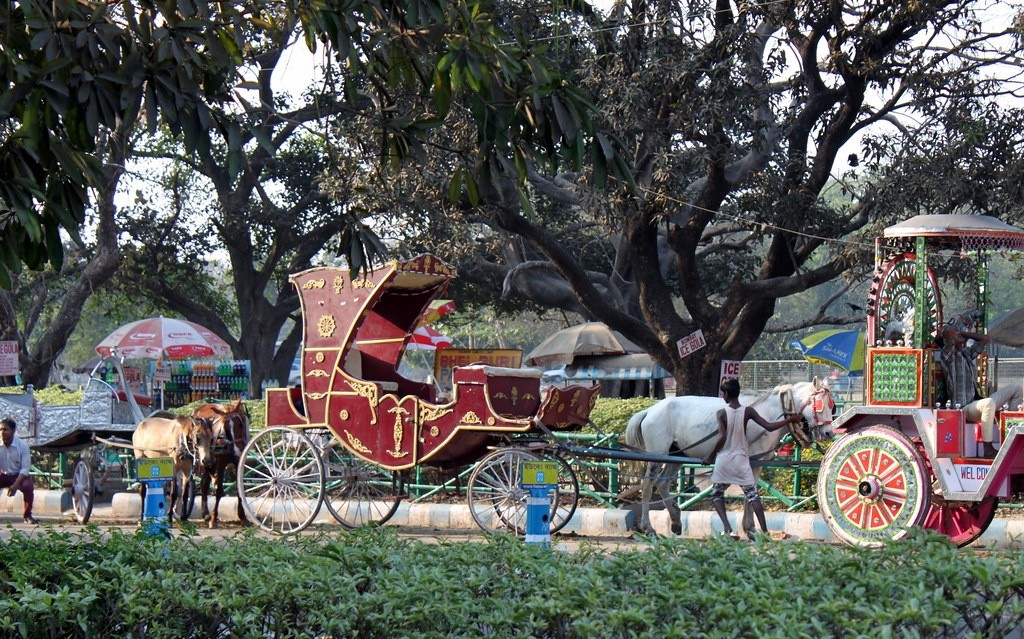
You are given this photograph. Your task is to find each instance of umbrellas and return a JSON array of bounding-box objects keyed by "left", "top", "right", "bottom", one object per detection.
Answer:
[
  {"left": 519, "top": 320, "right": 655, "bottom": 401},
  {"left": 402, "top": 296, "right": 455, "bottom": 358},
  {"left": 788, "top": 328, "right": 867, "bottom": 400},
  {"left": 94, "top": 312, "right": 234, "bottom": 415}
]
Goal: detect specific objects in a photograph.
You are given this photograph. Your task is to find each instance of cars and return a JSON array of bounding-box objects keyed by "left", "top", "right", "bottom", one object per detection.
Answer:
[{"left": 283, "top": 363, "right": 301, "bottom": 384}]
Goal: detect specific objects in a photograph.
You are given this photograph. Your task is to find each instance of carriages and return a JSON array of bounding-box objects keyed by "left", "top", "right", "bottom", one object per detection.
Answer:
[
  {"left": 30, "top": 397, "right": 254, "bottom": 531},
  {"left": 235, "top": 252, "right": 837, "bottom": 543},
  {"left": 815, "top": 215, "right": 1024, "bottom": 559}
]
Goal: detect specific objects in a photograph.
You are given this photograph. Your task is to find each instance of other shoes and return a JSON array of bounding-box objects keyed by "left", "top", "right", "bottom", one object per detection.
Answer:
[{"left": 24, "top": 517, "right": 38, "bottom": 524}]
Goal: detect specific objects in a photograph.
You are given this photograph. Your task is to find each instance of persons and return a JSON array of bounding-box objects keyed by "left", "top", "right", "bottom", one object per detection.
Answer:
[
  {"left": 701, "top": 381, "right": 804, "bottom": 538},
  {"left": 0, "top": 418, "right": 40, "bottom": 527},
  {"left": 940, "top": 322, "right": 1024, "bottom": 460}
]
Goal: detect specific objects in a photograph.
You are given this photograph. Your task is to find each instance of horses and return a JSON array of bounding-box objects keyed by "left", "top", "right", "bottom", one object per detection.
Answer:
[
  {"left": 625, "top": 376, "right": 837, "bottom": 542},
  {"left": 189, "top": 398, "right": 253, "bottom": 528},
  {"left": 131, "top": 410, "right": 214, "bottom": 528}
]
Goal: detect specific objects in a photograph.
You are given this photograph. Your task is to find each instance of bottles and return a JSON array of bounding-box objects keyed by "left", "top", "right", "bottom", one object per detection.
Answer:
[{"left": 98, "top": 358, "right": 279, "bottom": 414}]
